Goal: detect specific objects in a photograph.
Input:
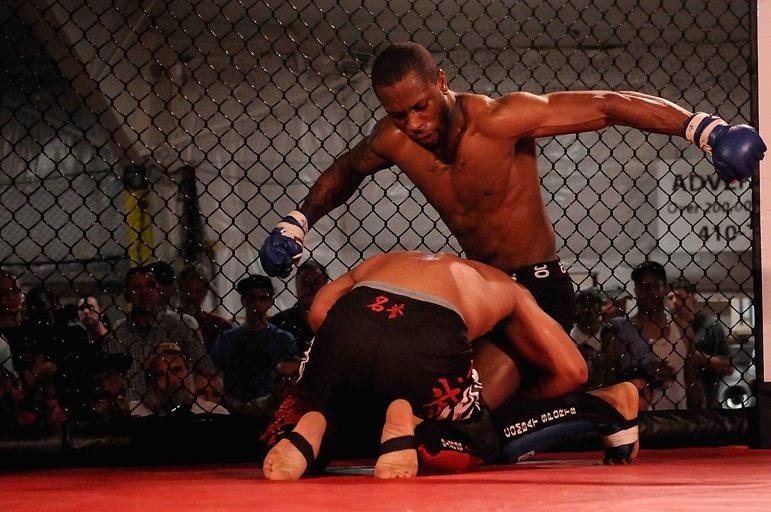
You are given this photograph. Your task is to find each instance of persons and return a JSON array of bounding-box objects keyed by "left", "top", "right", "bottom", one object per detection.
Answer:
[
  {"left": 262, "top": 251, "right": 589, "bottom": 478},
  {"left": 257, "top": 40, "right": 768, "bottom": 467},
  {"left": 569, "top": 259, "right": 735, "bottom": 444},
  {"left": 0, "top": 256, "right": 329, "bottom": 429}
]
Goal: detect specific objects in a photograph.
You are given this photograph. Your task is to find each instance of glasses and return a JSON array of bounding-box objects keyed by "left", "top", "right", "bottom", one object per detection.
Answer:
[{"left": 245, "top": 295, "right": 268, "bottom": 302}]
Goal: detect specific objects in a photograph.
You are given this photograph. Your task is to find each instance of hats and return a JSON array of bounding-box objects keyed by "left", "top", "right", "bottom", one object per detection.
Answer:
[
  {"left": 575, "top": 286, "right": 632, "bottom": 306},
  {"left": 237, "top": 274, "right": 274, "bottom": 295},
  {"left": 632, "top": 261, "right": 667, "bottom": 284}
]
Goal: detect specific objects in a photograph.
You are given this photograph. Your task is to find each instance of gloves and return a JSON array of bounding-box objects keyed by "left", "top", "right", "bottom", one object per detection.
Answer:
[
  {"left": 684, "top": 111, "right": 767, "bottom": 182},
  {"left": 259, "top": 208, "right": 312, "bottom": 278}
]
